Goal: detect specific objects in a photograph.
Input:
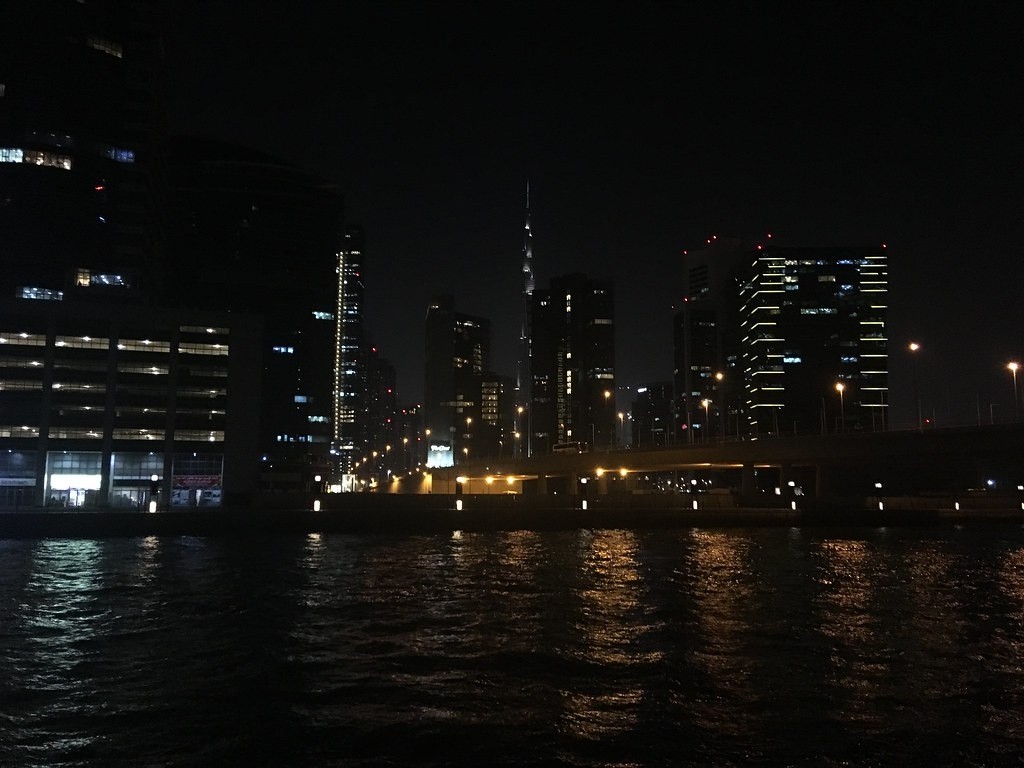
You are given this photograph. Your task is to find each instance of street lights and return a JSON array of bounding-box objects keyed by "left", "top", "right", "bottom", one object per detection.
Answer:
[
  {"left": 355, "top": 462, "right": 359, "bottom": 489},
  {"left": 363, "top": 457, "right": 366, "bottom": 490},
  {"left": 834, "top": 382, "right": 847, "bottom": 434},
  {"left": 715, "top": 371, "right": 726, "bottom": 440},
  {"left": 465, "top": 417, "right": 472, "bottom": 465},
  {"left": 1007, "top": 361, "right": 1020, "bottom": 424},
  {"left": 618, "top": 412, "right": 625, "bottom": 448},
  {"left": 908, "top": 340, "right": 922, "bottom": 428},
  {"left": 425, "top": 429, "right": 431, "bottom": 466},
  {"left": 403, "top": 437, "right": 408, "bottom": 477},
  {"left": 604, "top": 391, "right": 609, "bottom": 445},
  {"left": 372, "top": 451, "right": 378, "bottom": 485},
  {"left": 517, "top": 405, "right": 524, "bottom": 458},
  {"left": 385, "top": 445, "right": 390, "bottom": 485},
  {"left": 699, "top": 400, "right": 711, "bottom": 441},
  {"left": 462, "top": 448, "right": 468, "bottom": 465}
]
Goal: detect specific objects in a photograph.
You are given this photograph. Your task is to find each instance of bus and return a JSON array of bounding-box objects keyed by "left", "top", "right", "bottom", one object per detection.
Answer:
[{"left": 552, "top": 441, "right": 589, "bottom": 454}]
